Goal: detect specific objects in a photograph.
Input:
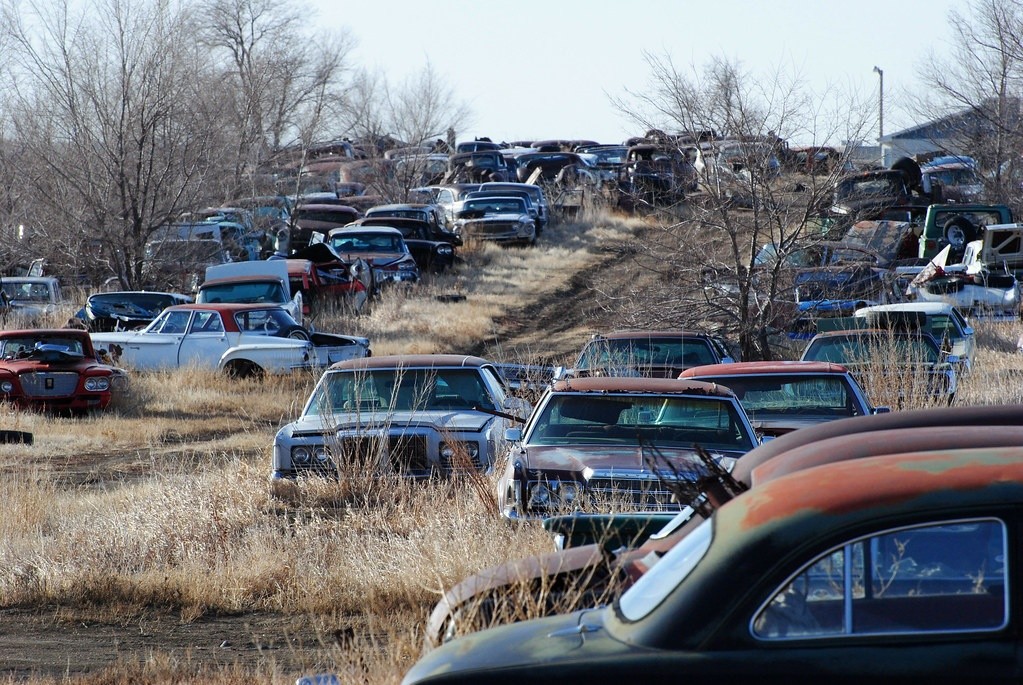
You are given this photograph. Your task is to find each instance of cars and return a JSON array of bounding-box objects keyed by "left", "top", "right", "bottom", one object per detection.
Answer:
[{"left": 2, "top": 126, "right": 1023, "bottom": 685}]
